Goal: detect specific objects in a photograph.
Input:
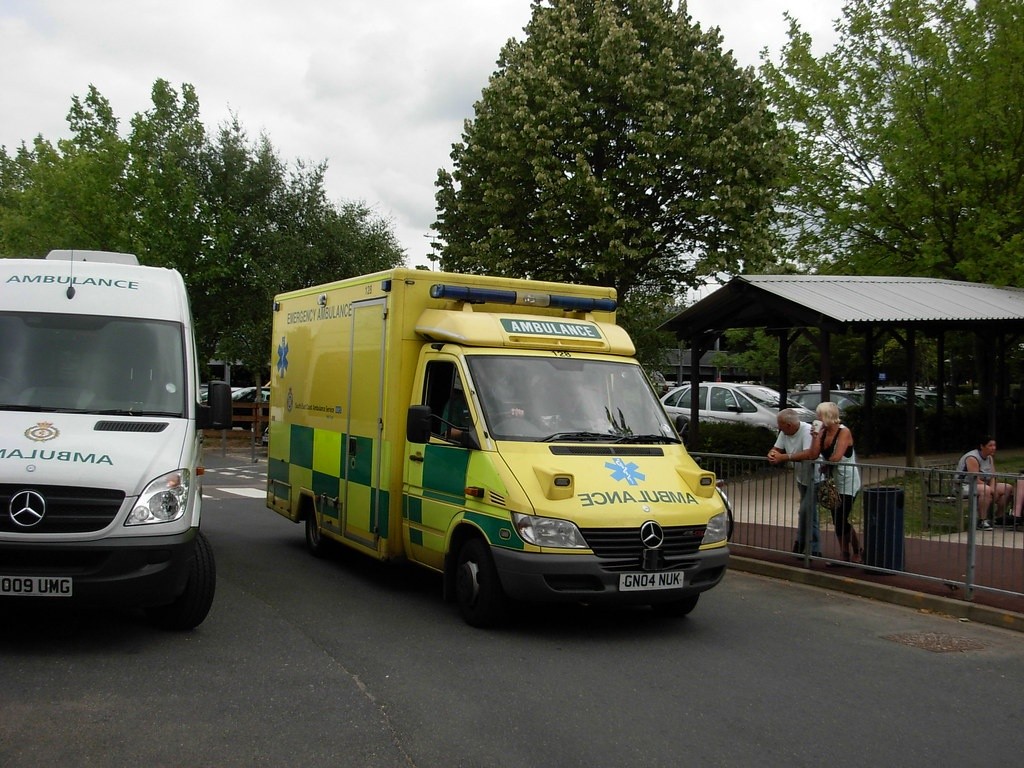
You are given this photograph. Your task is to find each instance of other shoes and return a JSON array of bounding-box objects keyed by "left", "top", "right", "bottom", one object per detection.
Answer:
[
  {"left": 852, "top": 548, "right": 863, "bottom": 563},
  {"left": 837, "top": 556, "right": 850, "bottom": 561}
]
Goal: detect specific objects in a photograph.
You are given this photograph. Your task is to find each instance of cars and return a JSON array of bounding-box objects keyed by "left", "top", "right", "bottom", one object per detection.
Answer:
[
  {"left": 658, "top": 382, "right": 847, "bottom": 441},
  {"left": 644, "top": 368, "right": 963, "bottom": 423},
  {"left": 196, "top": 381, "right": 270, "bottom": 445}
]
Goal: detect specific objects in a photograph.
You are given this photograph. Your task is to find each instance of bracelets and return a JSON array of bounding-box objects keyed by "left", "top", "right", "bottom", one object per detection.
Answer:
[{"left": 789, "top": 453, "right": 791, "bottom": 461}]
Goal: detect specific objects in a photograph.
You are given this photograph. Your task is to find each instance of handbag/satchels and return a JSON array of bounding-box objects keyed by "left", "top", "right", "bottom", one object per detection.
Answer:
[{"left": 815, "top": 464, "right": 842, "bottom": 510}]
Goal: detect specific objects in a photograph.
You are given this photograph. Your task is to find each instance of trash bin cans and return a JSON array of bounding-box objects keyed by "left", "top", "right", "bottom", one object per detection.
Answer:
[{"left": 862, "top": 486, "right": 907, "bottom": 576}]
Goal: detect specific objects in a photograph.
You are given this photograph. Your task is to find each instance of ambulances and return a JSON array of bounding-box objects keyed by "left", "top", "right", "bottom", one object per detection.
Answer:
[
  {"left": 265, "top": 269, "right": 735, "bottom": 628},
  {"left": 0, "top": 250, "right": 217, "bottom": 633}
]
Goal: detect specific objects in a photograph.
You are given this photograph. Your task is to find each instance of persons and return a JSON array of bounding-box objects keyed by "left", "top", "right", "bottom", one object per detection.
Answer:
[
  {"left": 441, "top": 366, "right": 524, "bottom": 440},
  {"left": 809, "top": 402, "right": 861, "bottom": 563},
  {"left": 952, "top": 436, "right": 1013, "bottom": 531},
  {"left": 767, "top": 409, "right": 824, "bottom": 556}
]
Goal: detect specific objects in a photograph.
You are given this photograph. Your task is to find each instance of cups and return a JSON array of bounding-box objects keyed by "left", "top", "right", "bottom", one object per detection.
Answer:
[{"left": 813, "top": 420, "right": 823, "bottom": 433}]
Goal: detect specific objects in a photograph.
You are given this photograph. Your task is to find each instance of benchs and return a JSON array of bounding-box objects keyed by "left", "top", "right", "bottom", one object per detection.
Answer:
[{"left": 927, "top": 466, "right": 980, "bottom": 533}]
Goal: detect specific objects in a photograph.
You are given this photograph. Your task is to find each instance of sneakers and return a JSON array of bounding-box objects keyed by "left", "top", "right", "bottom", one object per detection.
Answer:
[
  {"left": 994, "top": 517, "right": 1014, "bottom": 528},
  {"left": 977, "top": 519, "right": 993, "bottom": 530}
]
